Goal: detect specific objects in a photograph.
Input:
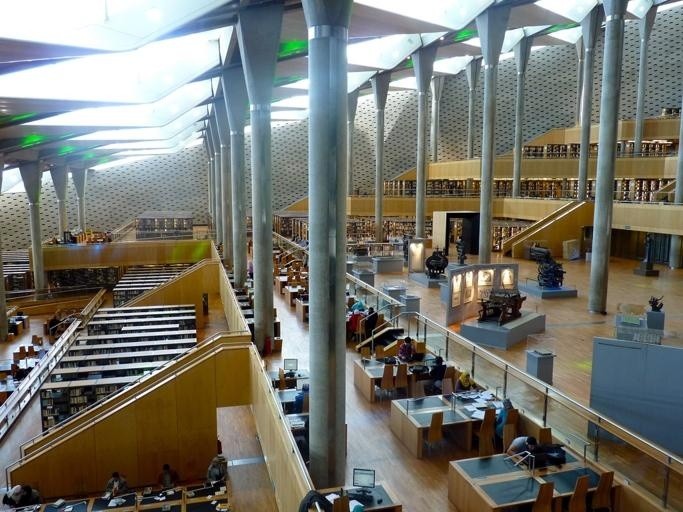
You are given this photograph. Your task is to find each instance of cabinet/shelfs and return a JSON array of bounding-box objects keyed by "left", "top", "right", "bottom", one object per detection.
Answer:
[
  {"left": 273, "top": 212, "right": 530, "bottom": 252},
  {"left": 39, "top": 264, "right": 198, "bottom": 437}
]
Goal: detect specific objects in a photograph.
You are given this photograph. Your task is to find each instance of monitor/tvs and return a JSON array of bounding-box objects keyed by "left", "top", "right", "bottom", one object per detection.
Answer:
[
  {"left": 620, "top": 315, "right": 641, "bottom": 326},
  {"left": 534, "top": 349, "right": 552, "bottom": 355},
  {"left": 457, "top": 389, "right": 504, "bottom": 420}
]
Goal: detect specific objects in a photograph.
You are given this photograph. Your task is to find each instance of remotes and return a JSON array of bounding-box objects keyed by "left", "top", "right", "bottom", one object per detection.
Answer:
[
  {"left": 298, "top": 374, "right": 301, "bottom": 377},
  {"left": 377, "top": 498, "right": 382, "bottom": 504}
]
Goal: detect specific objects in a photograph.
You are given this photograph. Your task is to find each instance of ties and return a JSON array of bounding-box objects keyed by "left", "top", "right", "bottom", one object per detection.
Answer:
[
  {"left": 284, "top": 359, "right": 298, "bottom": 374},
  {"left": 352, "top": 468, "right": 375, "bottom": 493}
]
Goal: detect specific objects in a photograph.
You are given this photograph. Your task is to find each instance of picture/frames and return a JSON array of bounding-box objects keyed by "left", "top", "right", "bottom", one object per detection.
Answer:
[
  {"left": 0, "top": 315, "right": 75, "bottom": 407},
  {"left": 184, "top": 496, "right": 229, "bottom": 512},
  {"left": 2, "top": 484, "right": 184, "bottom": 512},
  {"left": 184, "top": 481, "right": 228, "bottom": 501}
]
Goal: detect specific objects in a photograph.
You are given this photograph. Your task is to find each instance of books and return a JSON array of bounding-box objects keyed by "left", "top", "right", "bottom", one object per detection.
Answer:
[
  {"left": 620, "top": 315, "right": 641, "bottom": 326},
  {"left": 534, "top": 349, "right": 552, "bottom": 355},
  {"left": 24, "top": 498, "right": 73, "bottom": 512},
  {"left": 42, "top": 309, "right": 192, "bottom": 433},
  {"left": 102, "top": 487, "right": 152, "bottom": 506},
  {"left": 155, "top": 487, "right": 195, "bottom": 500},
  {"left": 215, "top": 486, "right": 229, "bottom": 512},
  {"left": 457, "top": 389, "right": 504, "bottom": 420},
  {"left": 114, "top": 290, "right": 138, "bottom": 306}
]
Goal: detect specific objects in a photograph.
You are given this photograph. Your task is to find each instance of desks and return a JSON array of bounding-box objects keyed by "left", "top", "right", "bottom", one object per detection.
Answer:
[
  {"left": 214, "top": 245, "right": 623, "bottom": 512},
  {"left": 184, "top": 496, "right": 229, "bottom": 512},
  {"left": 184, "top": 481, "right": 228, "bottom": 501},
  {"left": 0, "top": 315, "right": 75, "bottom": 407},
  {"left": 2, "top": 484, "right": 184, "bottom": 512}
]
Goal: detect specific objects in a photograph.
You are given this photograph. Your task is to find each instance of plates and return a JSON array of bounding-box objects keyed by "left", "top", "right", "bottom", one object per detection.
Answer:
[
  {"left": 273, "top": 212, "right": 530, "bottom": 252},
  {"left": 39, "top": 264, "right": 198, "bottom": 437}
]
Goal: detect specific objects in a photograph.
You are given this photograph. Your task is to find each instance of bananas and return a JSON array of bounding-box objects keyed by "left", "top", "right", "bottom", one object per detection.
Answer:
[
  {"left": 284, "top": 374, "right": 294, "bottom": 377},
  {"left": 348, "top": 493, "right": 373, "bottom": 504}
]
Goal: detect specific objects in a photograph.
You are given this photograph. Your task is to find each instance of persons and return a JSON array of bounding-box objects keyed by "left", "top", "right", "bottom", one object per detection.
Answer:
[
  {"left": 506, "top": 434, "right": 540, "bottom": 462},
  {"left": 428, "top": 355, "right": 448, "bottom": 396},
  {"left": 246, "top": 260, "right": 254, "bottom": 279},
  {"left": 17, "top": 486, "right": 40, "bottom": 506},
  {"left": 292, "top": 383, "right": 310, "bottom": 415},
  {"left": 363, "top": 307, "right": 379, "bottom": 336},
  {"left": 159, "top": 464, "right": 179, "bottom": 489},
  {"left": 208, "top": 458, "right": 227, "bottom": 480},
  {"left": 495, "top": 398, "right": 516, "bottom": 438},
  {"left": 346, "top": 308, "right": 364, "bottom": 343},
  {"left": 351, "top": 300, "right": 366, "bottom": 311},
  {"left": 50, "top": 314, "right": 61, "bottom": 336},
  {"left": 455, "top": 370, "right": 479, "bottom": 394},
  {"left": 108, "top": 472, "right": 127, "bottom": 495},
  {"left": 3, "top": 485, "right": 37, "bottom": 508},
  {"left": 398, "top": 336, "right": 417, "bottom": 361}
]
